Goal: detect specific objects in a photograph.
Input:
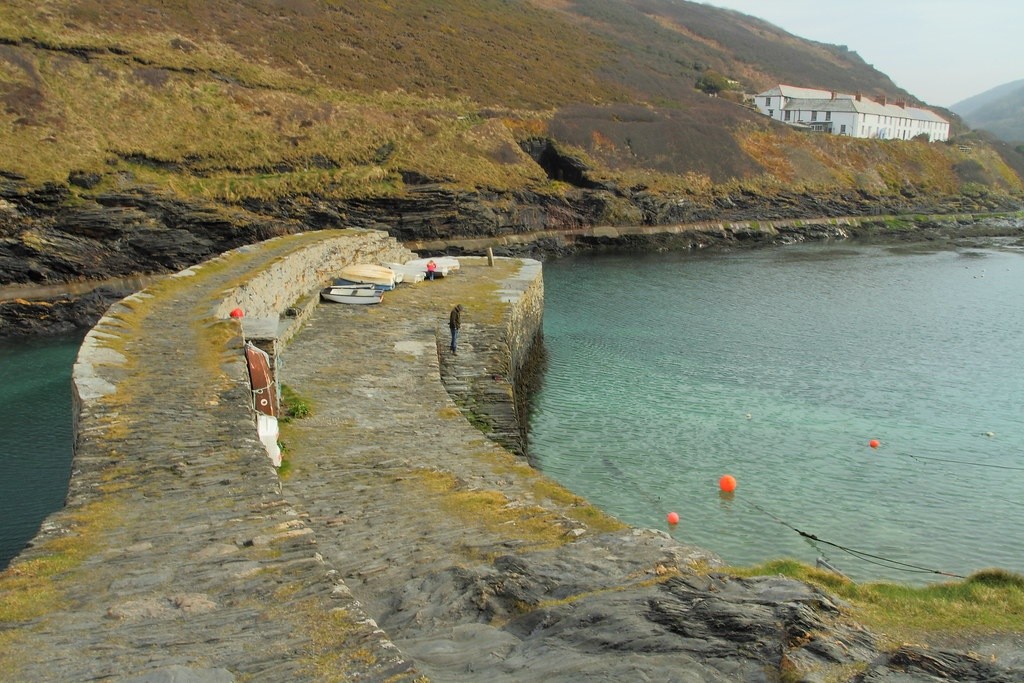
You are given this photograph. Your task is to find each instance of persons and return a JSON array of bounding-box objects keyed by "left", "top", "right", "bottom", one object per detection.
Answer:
[
  {"left": 427, "top": 260, "right": 436, "bottom": 282},
  {"left": 450, "top": 304, "right": 463, "bottom": 355}
]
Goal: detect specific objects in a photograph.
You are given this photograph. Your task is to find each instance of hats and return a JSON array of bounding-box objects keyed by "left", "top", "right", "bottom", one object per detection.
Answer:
[{"left": 455, "top": 304, "right": 462, "bottom": 312}]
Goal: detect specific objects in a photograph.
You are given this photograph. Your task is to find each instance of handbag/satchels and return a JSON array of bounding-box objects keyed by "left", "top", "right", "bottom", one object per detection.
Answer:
[{"left": 456, "top": 319, "right": 461, "bottom": 328}]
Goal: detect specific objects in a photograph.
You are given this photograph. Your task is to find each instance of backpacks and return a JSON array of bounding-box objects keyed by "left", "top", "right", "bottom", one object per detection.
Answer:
[{"left": 286, "top": 307, "right": 302, "bottom": 316}]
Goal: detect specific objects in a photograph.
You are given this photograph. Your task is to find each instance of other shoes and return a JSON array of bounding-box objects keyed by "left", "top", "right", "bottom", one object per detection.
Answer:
[
  {"left": 450, "top": 349, "right": 453, "bottom": 354},
  {"left": 452, "top": 351, "right": 459, "bottom": 356}
]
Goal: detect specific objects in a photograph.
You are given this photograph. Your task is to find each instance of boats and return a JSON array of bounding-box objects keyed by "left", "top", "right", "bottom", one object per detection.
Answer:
[
  {"left": 320, "top": 284, "right": 384, "bottom": 304},
  {"left": 383, "top": 257, "right": 460, "bottom": 282},
  {"left": 335, "top": 263, "right": 396, "bottom": 290},
  {"left": 244, "top": 340, "right": 280, "bottom": 418}
]
